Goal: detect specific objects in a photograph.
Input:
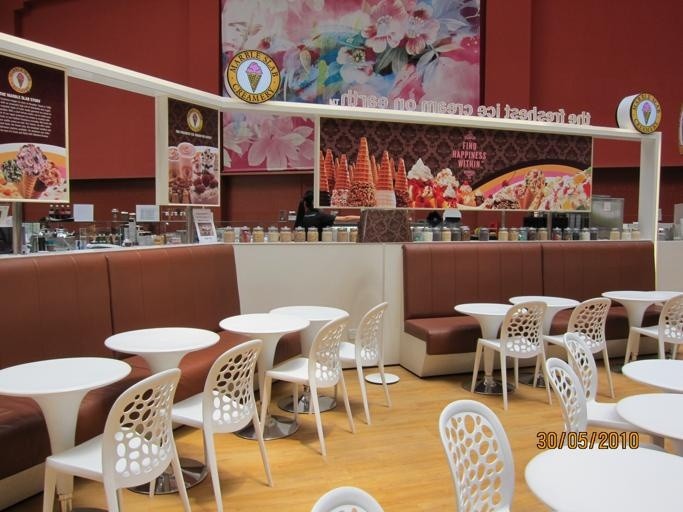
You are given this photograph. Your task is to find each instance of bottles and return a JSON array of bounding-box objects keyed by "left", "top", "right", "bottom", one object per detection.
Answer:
[
  {"left": 350, "top": 228, "right": 358, "bottom": 242},
  {"left": 322, "top": 226, "right": 332, "bottom": 242},
  {"left": 267, "top": 226, "right": 278, "bottom": 242},
  {"left": 111, "top": 208, "right": 119, "bottom": 220},
  {"left": 480, "top": 228, "right": 489, "bottom": 241},
  {"left": 572, "top": 228, "right": 579, "bottom": 240},
  {"left": 294, "top": 226, "right": 305, "bottom": 242},
  {"left": 610, "top": 228, "right": 620, "bottom": 241},
  {"left": 528, "top": 228, "right": 537, "bottom": 240},
  {"left": 518, "top": 227, "right": 526, "bottom": 241},
  {"left": 581, "top": 228, "right": 590, "bottom": 240},
  {"left": 414, "top": 226, "right": 423, "bottom": 240},
  {"left": 452, "top": 227, "right": 461, "bottom": 241},
  {"left": 631, "top": 228, "right": 640, "bottom": 240},
  {"left": 622, "top": 228, "right": 630, "bottom": 240},
  {"left": 424, "top": 227, "right": 433, "bottom": 241},
  {"left": 252, "top": 226, "right": 264, "bottom": 242},
  {"left": 223, "top": 225, "right": 235, "bottom": 243},
  {"left": 589, "top": 228, "right": 599, "bottom": 240},
  {"left": 96, "top": 234, "right": 123, "bottom": 245},
  {"left": 129, "top": 213, "right": 135, "bottom": 221},
  {"left": 461, "top": 226, "right": 470, "bottom": 241},
  {"left": 552, "top": 227, "right": 562, "bottom": 240},
  {"left": 563, "top": 227, "right": 573, "bottom": 240},
  {"left": 307, "top": 226, "right": 318, "bottom": 242},
  {"left": 490, "top": 228, "right": 497, "bottom": 240},
  {"left": 498, "top": 228, "right": 508, "bottom": 240},
  {"left": 280, "top": 225, "right": 291, "bottom": 242},
  {"left": 440, "top": 226, "right": 452, "bottom": 241},
  {"left": 510, "top": 228, "right": 518, "bottom": 240},
  {"left": 538, "top": 228, "right": 548, "bottom": 240},
  {"left": 54, "top": 204, "right": 60, "bottom": 218},
  {"left": 60, "top": 204, "right": 65, "bottom": 218},
  {"left": 337, "top": 228, "right": 348, "bottom": 242},
  {"left": 64, "top": 204, "right": 71, "bottom": 220},
  {"left": 48, "top": 205, "right": 54, "bottom": 219},
  {"left": 433, "top": 227, "right": 440, "bottom": 241},
  {"left": 121, "top": 212, "right": 128, "bottom": 221},
  {"left": 239, "top": 226, "right": 251, "bottom": 242}
]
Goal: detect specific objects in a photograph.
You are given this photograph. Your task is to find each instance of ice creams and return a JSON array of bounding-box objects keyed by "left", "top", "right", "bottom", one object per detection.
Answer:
[
  {"left": 436, "top": 169, "right": 457, "bottom": 208},
  {"left": 32, "top": 162, "right": 58, "bottom": 199},
  {"left": 377, "top": 151, "right": 397, "bottom": 209},
  {"left": 332, "top": 155, "right": 352, "bottom": 207},
  {"left": 18, "top": 145, "right": 47, "bottom": 198},
  {"left": 394, "top": 157, "right": 409, "bottom": 208},
  {"left": 347, "top": 137, "right": 377, "bottom": 206},
  {"left": 412, "top": 160, "right": 433, "bottom": 206},
  {"left": 320, "top": 151, "right": 329, "bottom": 205},
  {"left": 326, "top": 146, "right": 334, "bottom": 193}
]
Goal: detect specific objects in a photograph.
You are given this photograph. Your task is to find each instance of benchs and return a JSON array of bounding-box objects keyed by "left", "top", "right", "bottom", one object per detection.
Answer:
[
  {"left": 541, "top": 241, "right": 683, "bottom": 365},
  {"left": 105, "top": 243, "right": 303, "bottom": 435},
  {"left": 0, "top": 251, "right": 144, "bottom": 512},
  {"left": 397, "top": 241, "right": 578, "bottom": 378}
]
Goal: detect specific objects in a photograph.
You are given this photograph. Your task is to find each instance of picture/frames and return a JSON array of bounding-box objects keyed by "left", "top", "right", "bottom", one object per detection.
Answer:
[
  {"left": 156, "top": 97, "right": 220, "bottom": 206},
  {"left": 0, "top": 52, "right": 71, "bottom": 204},
  {"left": 218, "top": 0, "right": 487, "bottom": 179},
  {"left": 315, "top": 117, "right": 596, "bottom": 213}
]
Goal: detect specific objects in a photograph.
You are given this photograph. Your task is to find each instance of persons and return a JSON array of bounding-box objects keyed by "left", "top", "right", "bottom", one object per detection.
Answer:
[{"left": 293, "top": 189, "right": 334, "bottom": 229}]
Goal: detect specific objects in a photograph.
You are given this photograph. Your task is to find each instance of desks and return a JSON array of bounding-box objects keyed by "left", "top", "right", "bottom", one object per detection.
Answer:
[
  {"left": 602, "top": 291, "right": 676, "bottom": 373},
  {"left": 454, "top": 304, "right": 527, "bottom": 395},
  {"left": 269, "top": 306, "right": 348, "bottom": 414},
  {"left": 615, "top": 393, "right": 683, "bottom": 456},
  {"left": 523, "top": 442, "right": 683, "bottom": 512},
  {"left": 0, "top": 356, "right": 132, "bottom": 512},
  {"left": 219, "top": 313, "right": 310, "bottom": 442},
  {"left": 509, "top": 296, "right": 582, "bottom": 388},
  {"left": 103, "top": 327, "right": 221, "bottom": 495},
  {"left": 621, "top": 359, "right": 683, "bottom": 394}
]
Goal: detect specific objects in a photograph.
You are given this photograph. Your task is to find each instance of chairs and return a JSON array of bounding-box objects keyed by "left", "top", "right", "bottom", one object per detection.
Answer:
[
  {"left": 533, "top": 296, "right": 616, "bottom": 403},
  {"left": 45, "top": 368, "right": 191, "bottom": 512},
  {"left": 624, "top": 294, "right": 683, "bottom": 367},
  {"left": 564, "top": 332, "right": 665, "bottom": 449},
  {"left": 148, "top": 340, "right": 276, "bottom": 511},
  {"left": 260, "top": 314, "right": 356, "bottom": 456},
  {"left": 471, "top": 301, "right": 553, "bottom": 411},
  {"left": 544, "top": 357, "right": 667, "bottom": 455},
  {"left": 309, "top": 302, "right": 392, "bottom": 425},
  {"left": 309, "top": 487, "right": 385, "bottom": 512},
  {"left": 437, "top": 398, "right": 515, "bottom": 512}
]
